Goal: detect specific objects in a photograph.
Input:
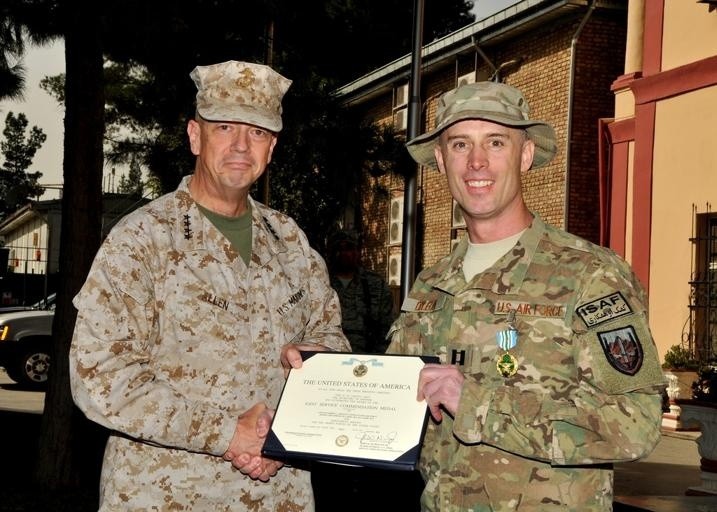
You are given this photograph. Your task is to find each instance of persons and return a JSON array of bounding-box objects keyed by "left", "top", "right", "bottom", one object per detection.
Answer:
[
  {"left": 222, "top": 81, "right": 668, "bottom": 512},
  {"left": 69, "top": 60, "right": 354, "bottom": 512}
]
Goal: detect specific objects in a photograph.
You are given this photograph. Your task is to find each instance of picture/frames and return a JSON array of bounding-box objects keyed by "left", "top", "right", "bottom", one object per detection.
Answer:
[{"left": 259, "top": 349, "right": 440, "bottom": 474}]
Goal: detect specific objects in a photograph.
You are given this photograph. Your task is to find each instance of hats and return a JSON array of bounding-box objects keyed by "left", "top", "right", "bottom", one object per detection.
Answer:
[
  {"left": 189, "top": 60, "right": 294, "bottom": 132},
  {"left": 406, "top": 81, "right": 557, "bottom": 172}
]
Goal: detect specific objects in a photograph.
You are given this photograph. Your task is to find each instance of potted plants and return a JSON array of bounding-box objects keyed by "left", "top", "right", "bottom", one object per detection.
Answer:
[{"left": 659, "top": 344, "right": 715, "bottom": 495}]
